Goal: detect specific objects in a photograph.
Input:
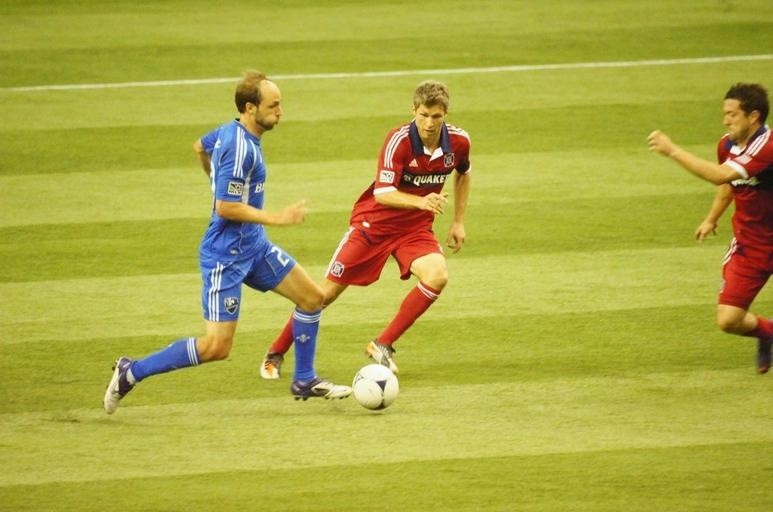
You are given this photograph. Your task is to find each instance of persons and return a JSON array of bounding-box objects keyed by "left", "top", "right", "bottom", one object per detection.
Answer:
[
  {"left": 104, "top": 69, "right": 353, "bottom": 414},
  {"left": 259, "top": 80, "right": 472, "bottom": 378},
  {"left": 646, "top": 80, "right": 773, "bottom": 373}
]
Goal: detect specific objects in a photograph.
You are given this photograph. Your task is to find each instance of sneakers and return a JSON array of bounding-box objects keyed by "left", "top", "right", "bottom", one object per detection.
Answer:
[
  {"left": 365, "top": 338, "right": 398, "bottom": 373},
  {"left": 291, "top": 376, "right": 353, "bottom": 401},
  {"left": 103, "top": 357, "right": 133, "bottom": 414},
  {"left": 758, "top": 338, "right": 772, "bottom": 373},
  {"left": 261, "top": 350, "right": 284, "bottom": 379}
]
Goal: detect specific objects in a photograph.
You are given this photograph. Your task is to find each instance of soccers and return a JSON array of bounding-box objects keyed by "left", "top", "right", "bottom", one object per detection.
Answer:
[{"left": 352, "top": 364, "right": 399, "bottom": 410}]
